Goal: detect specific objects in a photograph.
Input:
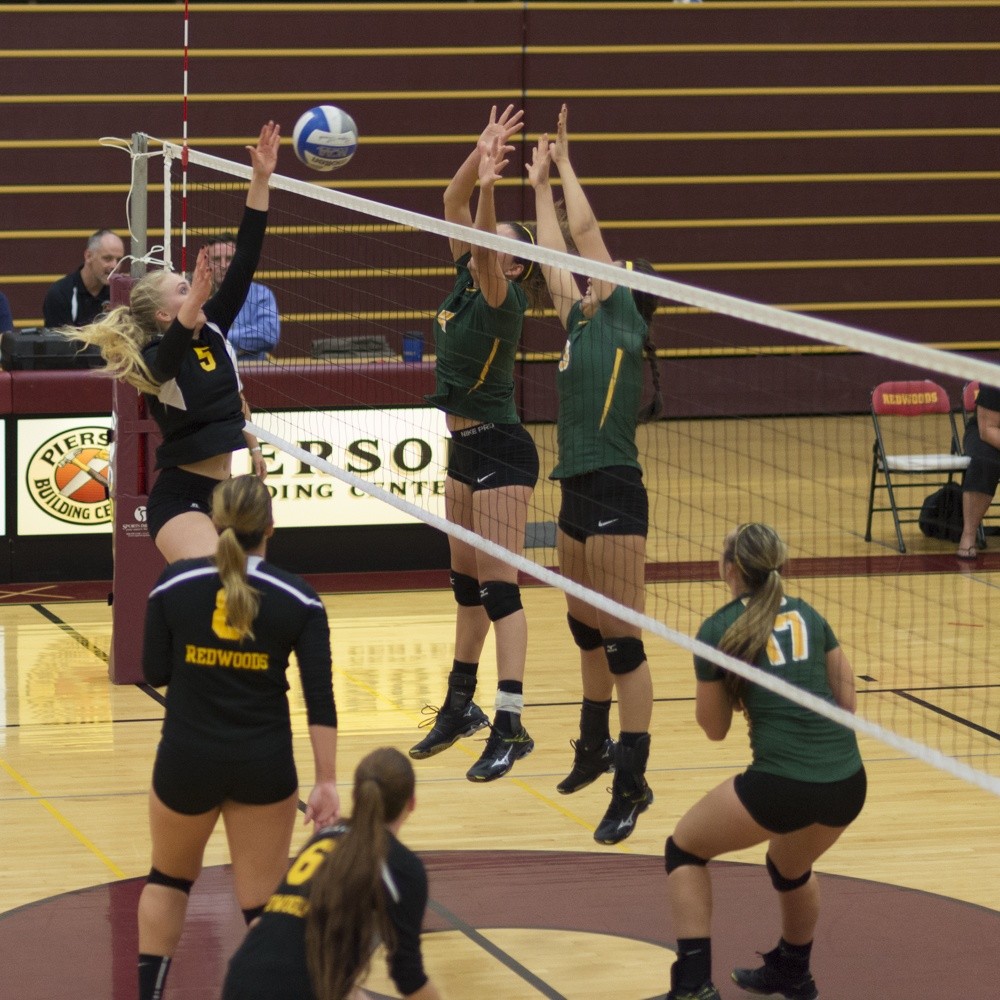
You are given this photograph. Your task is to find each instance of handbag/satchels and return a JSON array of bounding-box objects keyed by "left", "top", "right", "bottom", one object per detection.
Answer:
[{"left": 918, "top": 481, "right": 964, "bottom": 544}]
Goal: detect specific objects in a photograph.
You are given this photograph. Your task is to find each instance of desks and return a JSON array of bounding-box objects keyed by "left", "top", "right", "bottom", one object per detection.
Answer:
[{"left": 0, "top": 360, "right": 451, "bottom": 585}]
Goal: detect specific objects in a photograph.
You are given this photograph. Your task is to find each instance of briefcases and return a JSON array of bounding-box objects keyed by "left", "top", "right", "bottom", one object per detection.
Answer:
[{"left": 0, "top": 328, "right": 107, "bottom": 372}]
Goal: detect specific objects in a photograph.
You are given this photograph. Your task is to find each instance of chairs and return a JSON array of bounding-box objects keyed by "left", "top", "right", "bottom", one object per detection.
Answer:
[{"left": 862, "top": 379, "right": 1000, "bottom": 553}]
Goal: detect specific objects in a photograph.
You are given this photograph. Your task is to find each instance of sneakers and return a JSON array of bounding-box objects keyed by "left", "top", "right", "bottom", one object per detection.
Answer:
[
  {"left": 555, "top": 736, "right": 618, "bottom": 794},
  {"left": 666, "top": 982, "right": 722, "bottom": 1000},
  {"left": 465, "top": 716, "right": 535, "bottom": 783},
  {"left": 409, "top": 699, "right": 490, "bottom": 760},
  {"left": 730, "top": 948, "right": 819, "bottom": 1000},
  {"left": 593, "top": 774, "right": 653, "bottom": 845}
]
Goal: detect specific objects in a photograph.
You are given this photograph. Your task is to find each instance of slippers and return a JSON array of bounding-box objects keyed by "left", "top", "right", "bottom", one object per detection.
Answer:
[{"left": 956, "top": 545, "right": 978, "bottom": 559}]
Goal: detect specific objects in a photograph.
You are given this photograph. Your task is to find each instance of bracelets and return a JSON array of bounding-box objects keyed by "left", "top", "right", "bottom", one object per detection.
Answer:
[{"left": 249, "top": 446, "right": 262, "bottom": 456}]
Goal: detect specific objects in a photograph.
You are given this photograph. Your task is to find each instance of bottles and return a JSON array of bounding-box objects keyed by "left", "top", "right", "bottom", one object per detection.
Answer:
[{"left": 401, "top": 331, "right": 424, "bottom": 362}]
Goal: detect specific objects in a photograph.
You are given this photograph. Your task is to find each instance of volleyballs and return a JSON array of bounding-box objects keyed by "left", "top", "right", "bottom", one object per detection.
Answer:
[{"left": 292, "top": 105, "right": 358, "bottom": 172}]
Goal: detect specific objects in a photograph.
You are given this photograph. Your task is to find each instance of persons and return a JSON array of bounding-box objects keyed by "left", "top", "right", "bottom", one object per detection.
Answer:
[
  {"left": 200, "top": 232, "right": 280, "bottom": 362},
  {"left": 41, "top": 120, "right": 281, "bottom": 565},
  {"left": 957, "top": 385, "right": 1000, "bottom": 560},
  {"left": 524, "top": 104, "right": 663, "bottom": 845},
  {"left": 408, "top": 104, "right": 546, "bottom": 782},
  {"left": 220, "top": 748, "right": 443, "bottom": 1000},
  {"left": 42, "top": 229, "right": 125, "bottom": 328},
  {"left": 665, "top": 521, "right": 866, "bottom": 1000},
  {"left": 0, "top": 291, "right": 14, "bottom": 350},
  {"left": 137, "top": 474, "right": 340, "bottom": 1000}
]
